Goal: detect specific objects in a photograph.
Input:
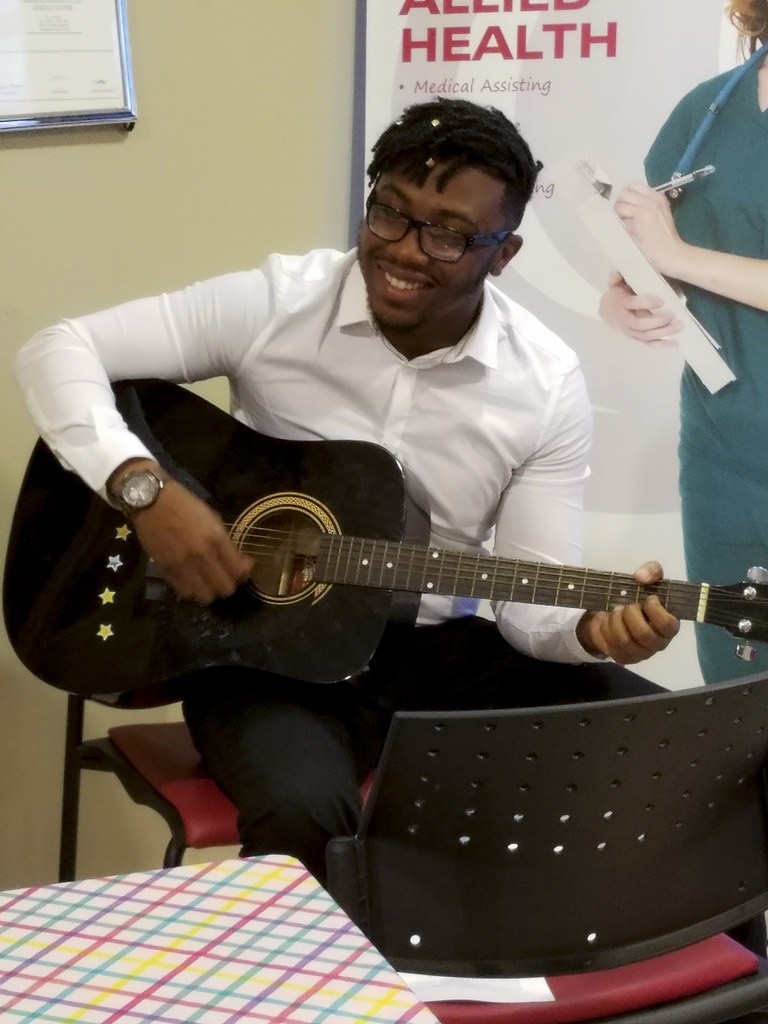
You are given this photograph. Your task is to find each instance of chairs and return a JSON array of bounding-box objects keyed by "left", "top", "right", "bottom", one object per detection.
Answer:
[
  {"left": 399, "top": 934, "right": 768, "bottom": 1024},
  {"left": 59, "top": 692, "right": 376, "bottom": 882}
]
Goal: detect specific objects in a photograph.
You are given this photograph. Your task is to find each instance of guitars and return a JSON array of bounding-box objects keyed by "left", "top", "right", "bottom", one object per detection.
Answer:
[{"left": 0, "top": 376, "right": 768, "bottom": 713}]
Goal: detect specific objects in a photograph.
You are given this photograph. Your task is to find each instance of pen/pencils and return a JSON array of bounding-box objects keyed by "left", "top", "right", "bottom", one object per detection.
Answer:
[{"left": 653, "top": 163, "right": 716, "bottom": 193}]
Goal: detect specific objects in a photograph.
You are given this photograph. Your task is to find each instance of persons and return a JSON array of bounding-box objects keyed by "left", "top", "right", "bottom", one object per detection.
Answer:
[
  {"left": 15, "top": 95, "right": 681, "bottom": 859},
  {"left": 594, "top": 0, "right": 768, "bottom": 682}
]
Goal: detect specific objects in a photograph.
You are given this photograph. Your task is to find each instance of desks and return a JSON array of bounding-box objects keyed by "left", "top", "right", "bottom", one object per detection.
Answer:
[{"left": 0, "top": 853, "right": 440, "bottom": 1024}]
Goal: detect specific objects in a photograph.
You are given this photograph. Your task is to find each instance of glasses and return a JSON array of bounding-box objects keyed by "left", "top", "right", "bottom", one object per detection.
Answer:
[{"left": 364, "top": 169, "right": 512, "bottom": 264}]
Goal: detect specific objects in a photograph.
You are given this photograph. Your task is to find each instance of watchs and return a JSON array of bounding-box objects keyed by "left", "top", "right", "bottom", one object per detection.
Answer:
[{"left": 119, "top": 467, "right": 172, "bottom": 522}]
